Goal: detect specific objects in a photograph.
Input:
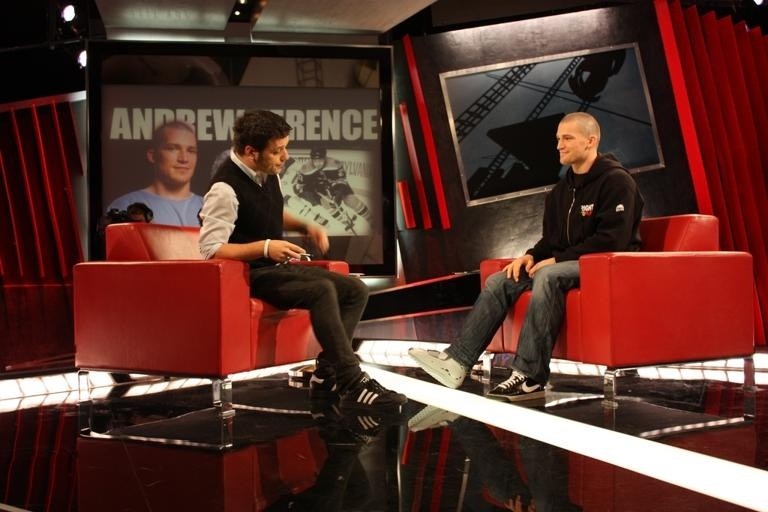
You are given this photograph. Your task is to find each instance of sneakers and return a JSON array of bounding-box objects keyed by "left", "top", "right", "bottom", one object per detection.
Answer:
[
  {"left": 309, "top": 370, "right": 338, "bottom": 400},
  {"left": 407, "top": 347, "right": 466, "bottom": 388},
  {"left": 485, "top": 371, "right": 546, "bottom": 404},
  {"left": 337, "top": 371, "right": 409, "bottom": 411}
]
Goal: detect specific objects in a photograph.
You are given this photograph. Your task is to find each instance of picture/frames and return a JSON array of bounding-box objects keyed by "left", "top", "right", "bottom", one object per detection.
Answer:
[{"left": 438, "top": 41, "right": 665, "bottom": 205}]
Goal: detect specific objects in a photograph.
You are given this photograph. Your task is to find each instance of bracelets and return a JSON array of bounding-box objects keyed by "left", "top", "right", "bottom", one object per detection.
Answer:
[{"left": 263, "top": 238, "right": 271, "bottom": 258}]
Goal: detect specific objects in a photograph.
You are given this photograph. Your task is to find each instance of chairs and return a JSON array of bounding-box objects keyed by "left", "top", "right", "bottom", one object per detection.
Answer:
[
  {"left": 477, "top": 212, "right": 755, "bottom": 412},
  {"left": 71, "top": 222, "right": 350, "bottom": 419}
]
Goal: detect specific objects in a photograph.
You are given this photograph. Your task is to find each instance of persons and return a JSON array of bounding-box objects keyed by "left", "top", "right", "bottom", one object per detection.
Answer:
[
  {"left": 107, "top": 119, "right": 205, "bottom": 228},
  {"left": 409, "top": 111, "right": 648, "bottom": 402},
  {"left": 279, "top": 154, "right": 329, "bottom": 227},
  {"left": 196, "top": 104, "right": 410, "bottom": 413},
  {"left": 265, "top": 411, "right": 384, "bottom": 512},
  {"left": 406, "top": 404, "right": 583, "bottom": 511},
  {"left": 292, "top": 145, "right": 371, "bottom": 226}
]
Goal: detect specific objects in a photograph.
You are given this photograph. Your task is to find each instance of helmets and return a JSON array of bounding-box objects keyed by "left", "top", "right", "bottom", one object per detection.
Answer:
[{"left": 310, "top": 148, "right": 328, "bottom": 170}]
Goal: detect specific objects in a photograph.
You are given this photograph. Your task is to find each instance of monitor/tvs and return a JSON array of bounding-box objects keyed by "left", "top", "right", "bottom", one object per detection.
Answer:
[{"left": 85, "top": 38, "right": 399, "bottom": 301}]
